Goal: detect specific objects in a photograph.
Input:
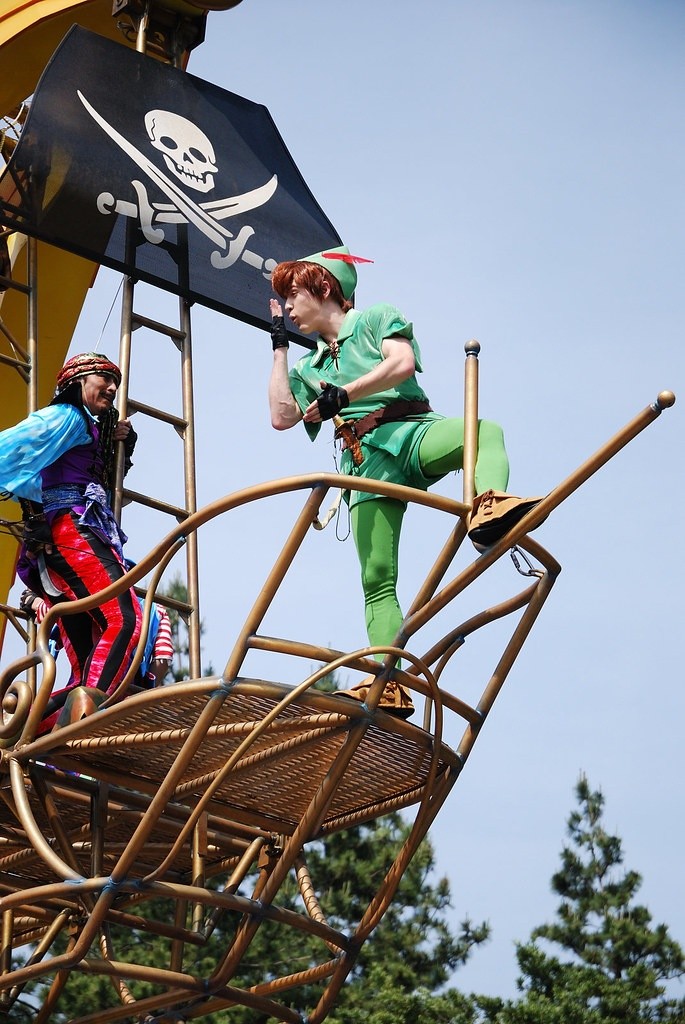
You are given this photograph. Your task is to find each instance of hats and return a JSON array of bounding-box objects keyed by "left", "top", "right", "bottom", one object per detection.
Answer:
[
  {"left": 56, "top": 353, "right": 122, "bottom": 392},
  {"left": 297, "top": 246, "right": 374, "bottom": 300}
]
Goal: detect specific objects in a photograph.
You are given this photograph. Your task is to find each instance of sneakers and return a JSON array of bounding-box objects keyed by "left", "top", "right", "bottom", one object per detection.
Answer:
[
  {"left": 332, "top": 675, "right": 415, "bottom": 719},
  {"left": 469, "top": 489, "right": 550, "bottom": 545}
]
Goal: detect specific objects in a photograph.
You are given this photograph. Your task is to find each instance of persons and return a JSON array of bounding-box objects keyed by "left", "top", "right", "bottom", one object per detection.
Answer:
[
  {"left": 267, "top": 245, "right": 549, "bottom": 720},
  {"left": 0, "top": 352, "right": 171, "bottom": 750}
]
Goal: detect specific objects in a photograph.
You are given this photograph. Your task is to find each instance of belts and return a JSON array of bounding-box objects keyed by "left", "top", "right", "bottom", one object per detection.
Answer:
[{"left": 341, "top": 401, "right": 434, "bottom": 451}]
